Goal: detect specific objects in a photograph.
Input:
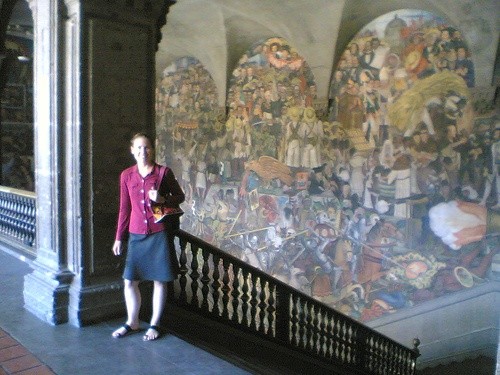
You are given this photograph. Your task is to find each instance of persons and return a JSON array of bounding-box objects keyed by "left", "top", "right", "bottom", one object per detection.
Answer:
[{"left": 110, "top": 131, "right": 185, "bottom": 343}]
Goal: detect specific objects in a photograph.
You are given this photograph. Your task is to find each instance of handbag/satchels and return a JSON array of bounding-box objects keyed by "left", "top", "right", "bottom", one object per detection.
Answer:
[{"left": 148, "top": 166, "right": 184, "bottom": 224}]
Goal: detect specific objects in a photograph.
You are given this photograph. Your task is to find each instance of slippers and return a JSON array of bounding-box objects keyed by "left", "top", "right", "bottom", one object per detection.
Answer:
[
  {"left": 111, "top": 323, "right": 142, "bottom": 340},
  {"left": 142, "top": 326, "right": 166, "bottom": 341}
]
